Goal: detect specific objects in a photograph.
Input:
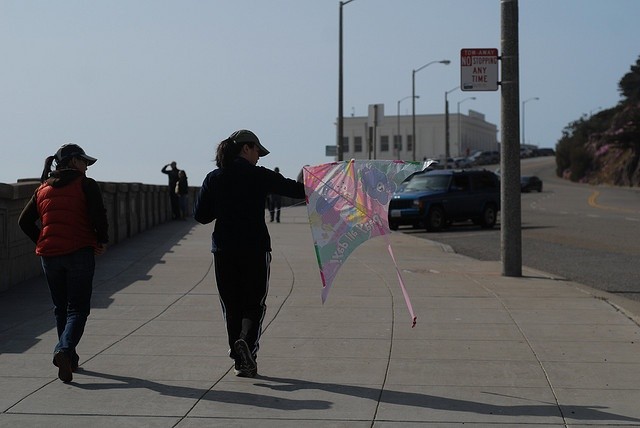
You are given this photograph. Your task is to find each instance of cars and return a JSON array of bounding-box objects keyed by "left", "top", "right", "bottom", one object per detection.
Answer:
[
  {"left": 520, "top": 145, "right": 554, "bottom": 158},
  {"left": 428, "top": 156, "right": 475, "bottom": 168},
  {"left": 520, "top": 175, "right": 543, "bottom": 192}
]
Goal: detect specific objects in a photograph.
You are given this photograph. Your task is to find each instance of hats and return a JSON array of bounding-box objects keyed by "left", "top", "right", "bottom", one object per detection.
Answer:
[
  {"left": 56, "top": 143, "right": 97, "bottom": 166},
  {"left": 230, "top": 130, "right": 270, "bottom": 157}
]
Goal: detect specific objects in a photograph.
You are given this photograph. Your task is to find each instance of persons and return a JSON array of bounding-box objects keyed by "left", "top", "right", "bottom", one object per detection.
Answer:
[
  {"left": 175, "top": 170, "right": 189, "bottom": 222},
  {"left": 18, "top": 143, "right": 109, "bottom": 383},
  {"left": 194, "top": 129, "right": 306, "bottom": 379},
  {"left": 161, "top": 161, "right": 181, "bottom": 220},
  {"left": 269, "top": 168, "right": 281, "bottom": 222}
]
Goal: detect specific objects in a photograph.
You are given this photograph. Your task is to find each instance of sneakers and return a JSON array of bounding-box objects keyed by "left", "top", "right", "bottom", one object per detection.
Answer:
[
  {"left": 234, "top": 336, "right": 257, "bottom": 378},
  {"left": 71, "top": 349, "right": 79, "bottom": 368},
  {"left": 54, "top": 349, "right": 73, "bottom": 381}
]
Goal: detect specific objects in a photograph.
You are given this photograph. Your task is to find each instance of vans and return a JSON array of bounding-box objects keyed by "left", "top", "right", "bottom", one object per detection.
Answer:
[
  {"left": 387, "top": 167, "right": 500, "bottom": 231},
  {"left": 469, "top": 150, "right": 500, "bottom": 165}
]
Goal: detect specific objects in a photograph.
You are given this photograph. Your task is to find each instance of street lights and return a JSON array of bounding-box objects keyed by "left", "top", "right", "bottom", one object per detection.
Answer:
[
  {"left": 522, "top": 97, "right": 540, "bottom": 145},
  {"left": 457, "top": 96, "right": 476, "bottom": 155},
  {"left": 397, "top": 95, "right": 420, "bottom": 159},
  {"left": 412, "top": 59, "right": 451, "bottom": 159}
]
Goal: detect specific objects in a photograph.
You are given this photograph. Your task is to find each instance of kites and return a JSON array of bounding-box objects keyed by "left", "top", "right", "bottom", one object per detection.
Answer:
[{"left": 302, "top": 158, "right": 439, "bottom": 329}]
